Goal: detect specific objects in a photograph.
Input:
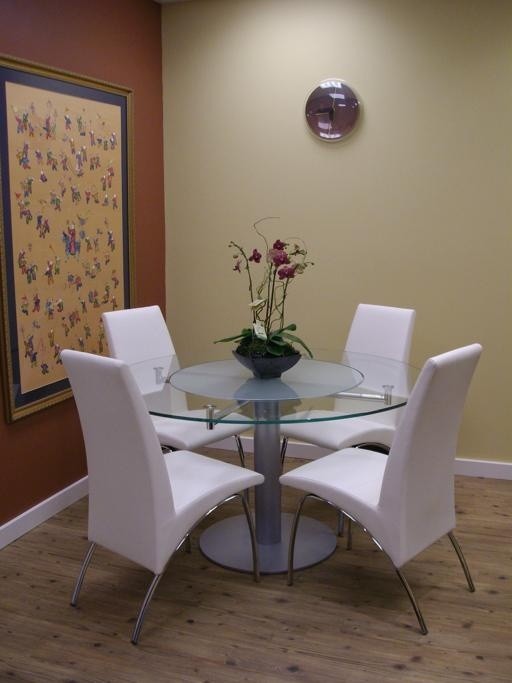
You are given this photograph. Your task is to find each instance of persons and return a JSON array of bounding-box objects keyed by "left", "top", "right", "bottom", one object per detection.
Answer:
[{"left": 9, "top": 93, "right": 122, "bottom": 374}]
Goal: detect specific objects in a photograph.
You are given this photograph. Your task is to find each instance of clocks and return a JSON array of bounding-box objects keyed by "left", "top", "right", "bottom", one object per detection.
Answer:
[{"left": 304, "top": 80, "right": 360, "bottom": 143}]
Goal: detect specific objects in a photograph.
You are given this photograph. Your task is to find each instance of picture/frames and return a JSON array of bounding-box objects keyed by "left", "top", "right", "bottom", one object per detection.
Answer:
[{"left": 1, "top": 53, "right": 138, "bottom": 425}]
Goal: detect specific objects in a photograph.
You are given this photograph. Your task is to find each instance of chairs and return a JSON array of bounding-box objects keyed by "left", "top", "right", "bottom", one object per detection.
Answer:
[
  {"left": 280, "top": 303, "right": 416, "bottom": 550},
  {"left": 277, "top": 343, "right": 483, "bottom": 635},
  {"left": 60, "top": 348, "right": 266, "bottom": 646},
  {"left": 101, "top": 306, "right": 254, "bottom": 556}
]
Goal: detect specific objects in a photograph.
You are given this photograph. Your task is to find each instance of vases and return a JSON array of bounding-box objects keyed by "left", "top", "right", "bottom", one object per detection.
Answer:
[{"left": 234, "top": 356, "right": 300, "bottom": 379}]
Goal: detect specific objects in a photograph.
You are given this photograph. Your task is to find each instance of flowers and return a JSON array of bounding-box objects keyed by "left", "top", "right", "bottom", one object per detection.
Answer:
[{"left": 213, "top": 216, "right": 315, "bottom": 358}]
